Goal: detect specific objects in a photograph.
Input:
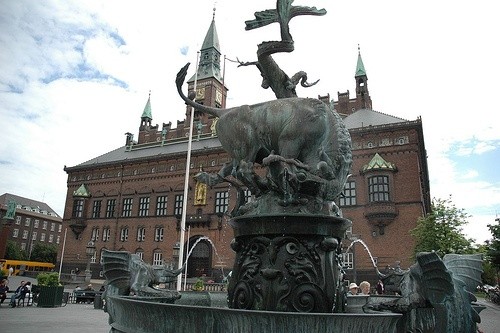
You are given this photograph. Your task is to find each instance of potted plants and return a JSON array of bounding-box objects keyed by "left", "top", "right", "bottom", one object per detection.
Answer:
[{"left": 32, "top": 270, "right": 64, "bottom": 308}]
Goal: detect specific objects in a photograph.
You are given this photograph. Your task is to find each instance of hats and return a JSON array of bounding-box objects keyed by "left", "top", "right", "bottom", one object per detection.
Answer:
[
  {"left": 88, "top": 284, "right": 92, "bottom": 287},
  {"left": 349, "top": 282, "right": 358, "bottom": 289}
]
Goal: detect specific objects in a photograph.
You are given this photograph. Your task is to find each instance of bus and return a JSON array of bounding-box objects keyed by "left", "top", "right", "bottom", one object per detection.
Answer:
[{"left": 0, "top": 259, "right": 55, "bottom": 277}]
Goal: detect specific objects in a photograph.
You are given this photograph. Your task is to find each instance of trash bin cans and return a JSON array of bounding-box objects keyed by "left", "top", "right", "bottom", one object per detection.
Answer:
[{"left": 94, "top": 295, "right": 104, "bottom": 309}]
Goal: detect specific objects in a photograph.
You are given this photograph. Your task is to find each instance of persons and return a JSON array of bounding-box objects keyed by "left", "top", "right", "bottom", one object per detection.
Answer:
[
  {"left": 375, "top": 281, "right": 383, "bottom": 296},
  {"left": 0, "top": 279, "right": 10, "bottom": 306},
  {"left": 84, "top": 284, "right": 95, "bottom": 298},
  {"left": 359, "top": 281, "right": 371, "bottom": 295},
  {"left": 71, "top": 268, "right": 79, "bottom": 280},
  {"left": 9, "top": 280, "right": 27, "bottom": 308},
  {"left": 9, "top": 266, "right": 14, "bottom": 276},
  {"left": 347, "top": 283, "right": 359, "bottom": 296},
  {"left": 74, "top": 285, "right": 83, "bottom": 298},
  {"left": 99, "top": 285, "right": 105, "bottom": 295}
]
